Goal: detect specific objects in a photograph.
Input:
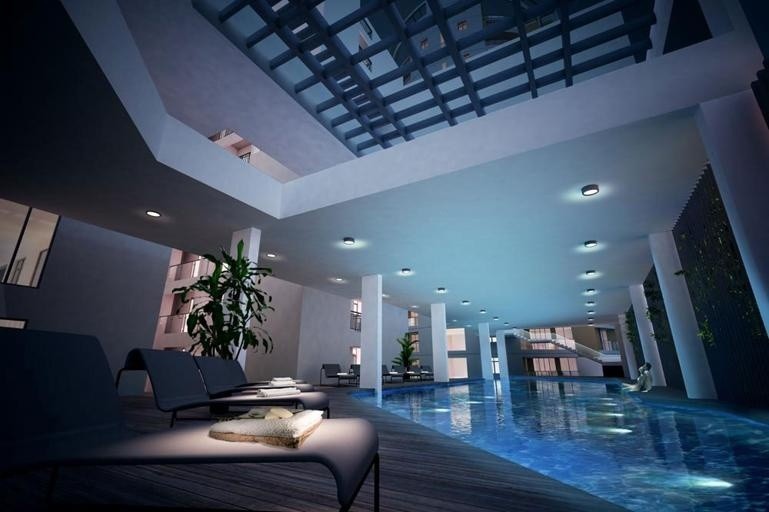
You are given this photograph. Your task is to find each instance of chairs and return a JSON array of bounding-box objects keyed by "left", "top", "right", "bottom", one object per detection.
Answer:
[
  {"left": 192, "top": 355, "right": 314, "bottom": 397},
  {"left": 219, "top": 358, "right": 307, "bottom": 387},
  {"left": 320, "top": 362, "right": 432, "bottom": 387},
  {"left": 1, "top": 325, "right": 379, "bottom": 512},
  {"left": 114, "top": 347, "right": 328, "bottom": 429}
]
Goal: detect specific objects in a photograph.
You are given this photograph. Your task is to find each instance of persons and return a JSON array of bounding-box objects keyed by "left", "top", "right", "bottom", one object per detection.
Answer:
[{"left": 621, "top": 362, "right": 652, "bottom": 393}]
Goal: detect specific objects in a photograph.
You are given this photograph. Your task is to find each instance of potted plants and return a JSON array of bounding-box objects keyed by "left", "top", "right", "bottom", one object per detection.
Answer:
[
  {"left": 167, "top": 235, "right": 279, "bottom": 415},
  {"left": 390, "top": 332, "right": 421, "bottom": 381}
]
